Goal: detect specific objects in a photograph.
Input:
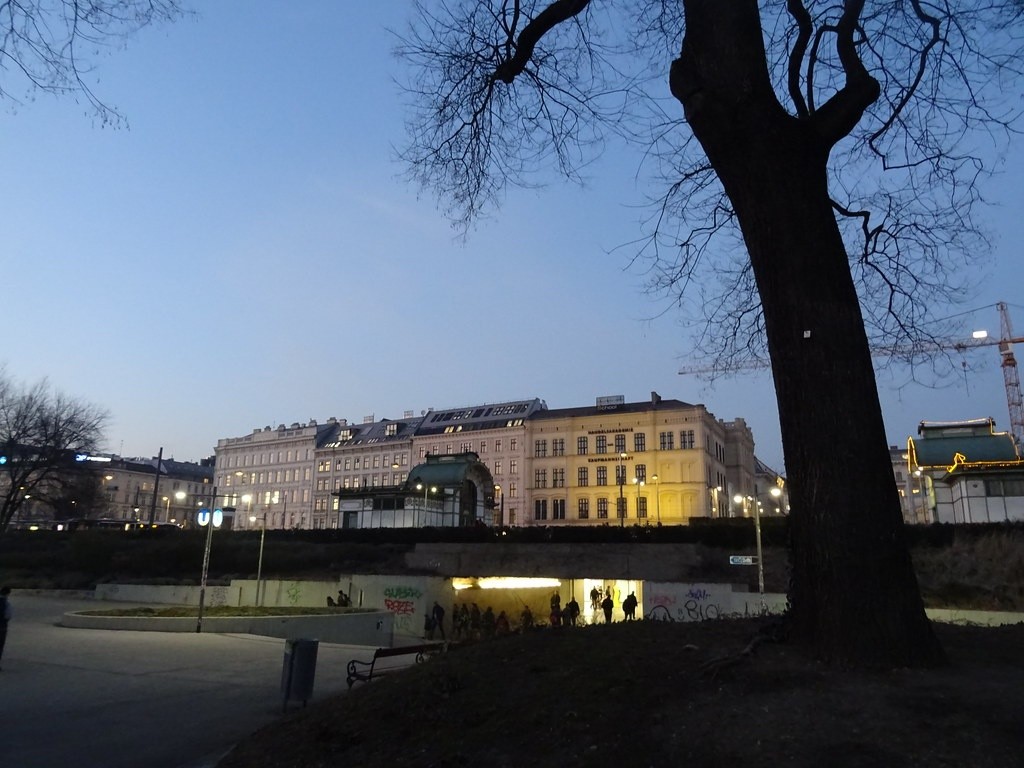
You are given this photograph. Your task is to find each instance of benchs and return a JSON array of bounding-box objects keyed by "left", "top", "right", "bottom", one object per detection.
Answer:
[{"left": 346, "top": 642, "right": 446, "bottom": 691}]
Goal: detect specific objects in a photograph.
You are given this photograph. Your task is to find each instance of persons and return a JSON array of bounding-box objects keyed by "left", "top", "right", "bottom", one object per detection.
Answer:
[
  {"left": 589, "top": 585, "right": 638, "bottom": 624},
  {"left": 424, "top": 603, "right": 509, "bottom": 641},
  {"left": 0, "top": 586, "right": 12, "bottom": 671},
  {"left": 548, "top": 590, "right": 580, "bottom": 627},
  {"left": 327, "top": 590, "right": 353, "bottom": 607},
  {"left": 520, "top": 605, "right": 534, "bottom": 628}
]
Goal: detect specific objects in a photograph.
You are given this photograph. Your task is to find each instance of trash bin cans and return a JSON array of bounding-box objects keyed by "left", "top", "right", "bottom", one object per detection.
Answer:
[{"left": 280, "top": 636, "right": 320, "bottom": 712}]
